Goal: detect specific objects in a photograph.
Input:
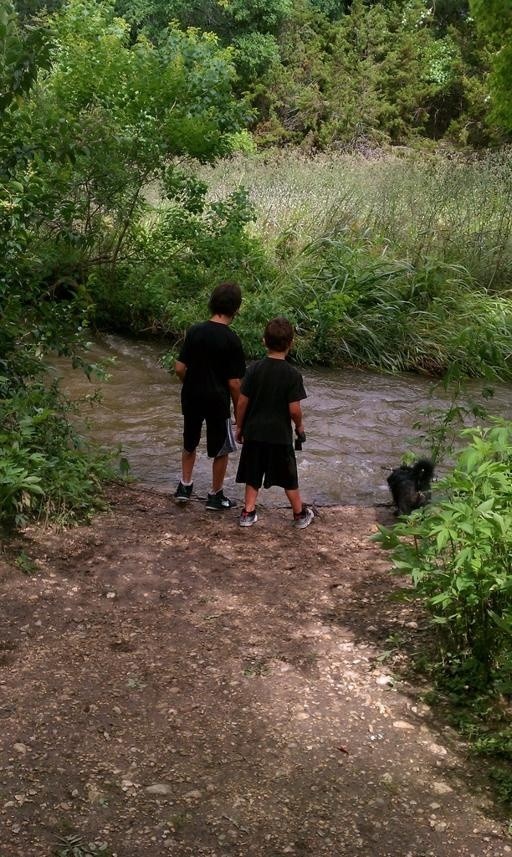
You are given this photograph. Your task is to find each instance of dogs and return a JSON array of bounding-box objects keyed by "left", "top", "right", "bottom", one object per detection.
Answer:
[{"left": 386, "top": 459, "right": 434, "bottom": 516}]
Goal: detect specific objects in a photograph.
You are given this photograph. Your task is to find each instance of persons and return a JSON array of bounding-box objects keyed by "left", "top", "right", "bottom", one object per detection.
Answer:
[
  {"left": 171, "top": 282, "right": 245, "bottom": 514},
  {"left": 234, "top": 315, "right": 317, "bottom": 528}
]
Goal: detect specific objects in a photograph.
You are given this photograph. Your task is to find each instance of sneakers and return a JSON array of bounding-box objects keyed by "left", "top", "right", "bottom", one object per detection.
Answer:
[
  {"left": 172, "top": 479, "right": 193, "bottom": 502},
  {"left": 239, "top": 508, "right": 258, "bottom": 527},
  {"left": 295, "top": 508, "right": 314, "bottom": 528},
  {"left": 205, "top": 489, "right": 239, "bottom": 510}
]
